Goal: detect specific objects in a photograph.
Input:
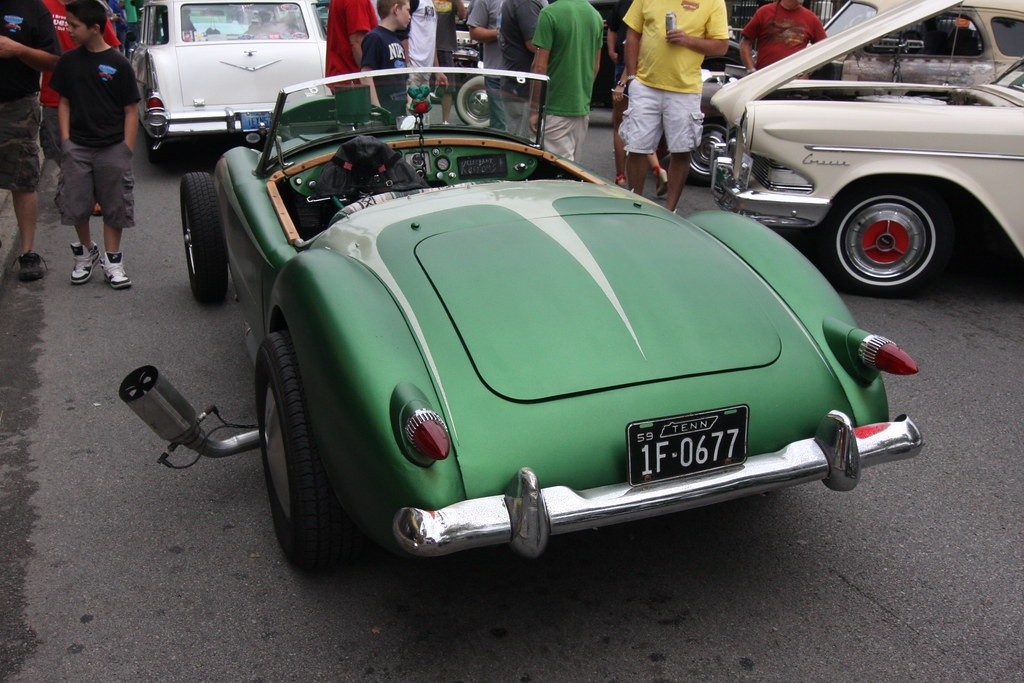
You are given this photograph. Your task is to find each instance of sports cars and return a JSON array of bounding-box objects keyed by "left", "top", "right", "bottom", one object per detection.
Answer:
[
  {"left": 106, "top": 63, "right": 925, "bottom": 580},
  {"left": 708, "top": 1, "right": 1023, "bottom": 298}
]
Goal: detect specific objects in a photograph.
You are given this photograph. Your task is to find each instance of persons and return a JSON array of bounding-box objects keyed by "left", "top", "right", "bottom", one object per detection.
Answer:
[
  {"left": 1, "top": 0, "right": 60, "bottom": 280},
  {"left": 41, "top": 0, "right": 138, "bottom": 218},
  {"left": 361, "top": 0, "right": 412, "bottom": 126},
  {"left": 621, "top": 0, "right": 728, "bottom": 212},
  {"left": 466, "top": 0, "right": 603, "bottom": 160},
  {"left": 738, "top": 0, "right": 827, "bottom": 75},
  {"left": 324, "top": 0, "right": 376, "bottom": 97},
  {"left": 49, "top": 0, "right": 142, "bottom": 288},
  {"left": 607, "top": 0, "right": 668, "bottom": 197},
  {"left": 396, "top": 0, "right": 449, "bottom": 109},
  {"left": 430, "top": 0, "right": 466, "bottom": 123}
]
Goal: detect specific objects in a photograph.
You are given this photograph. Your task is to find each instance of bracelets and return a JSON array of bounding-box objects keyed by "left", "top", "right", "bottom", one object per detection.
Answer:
[{"left": 747, "top": 69, "right": 756, "bottom": 73}]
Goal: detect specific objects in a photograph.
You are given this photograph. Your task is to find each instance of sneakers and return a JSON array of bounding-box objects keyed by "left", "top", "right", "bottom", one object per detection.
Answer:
[
  {"left": 18, "top": 253, "right": 43, "bottom": 280},
  {"left": 101, "top": 251, "right": 131, "bottom": 288},
  {"left": 71, "top": 241, "right": 101, "bottom": 283},
  {"left": 656, "top": 169, "right": 668, "bottom": 195},
  {"left": 615, "top": 175, "right": 627, "bottom": 186}
]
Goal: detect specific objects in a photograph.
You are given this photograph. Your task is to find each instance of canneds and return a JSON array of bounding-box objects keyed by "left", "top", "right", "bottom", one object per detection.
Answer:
[{"left": 665, "top": 13, "right": 677, "bottom": 32}]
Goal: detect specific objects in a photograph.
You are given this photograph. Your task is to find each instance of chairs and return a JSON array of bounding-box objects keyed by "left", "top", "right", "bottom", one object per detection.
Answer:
[
  {"left": 925, "top": 29, "right": 949, "bottom": 55},
  {"left": 310, "top": 135, "right": 428, "bottom": 229},
  {"left": 948, "top": 26, "right": 978, "bottom": 56}
]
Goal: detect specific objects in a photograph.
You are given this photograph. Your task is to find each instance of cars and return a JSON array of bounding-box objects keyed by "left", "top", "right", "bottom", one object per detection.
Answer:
[
  {"left": 124, "top": 1, "right": 337, "bottom": 162},
  {"left": 621, "top": 3, "right": 1024, "bottom": 191}
]
